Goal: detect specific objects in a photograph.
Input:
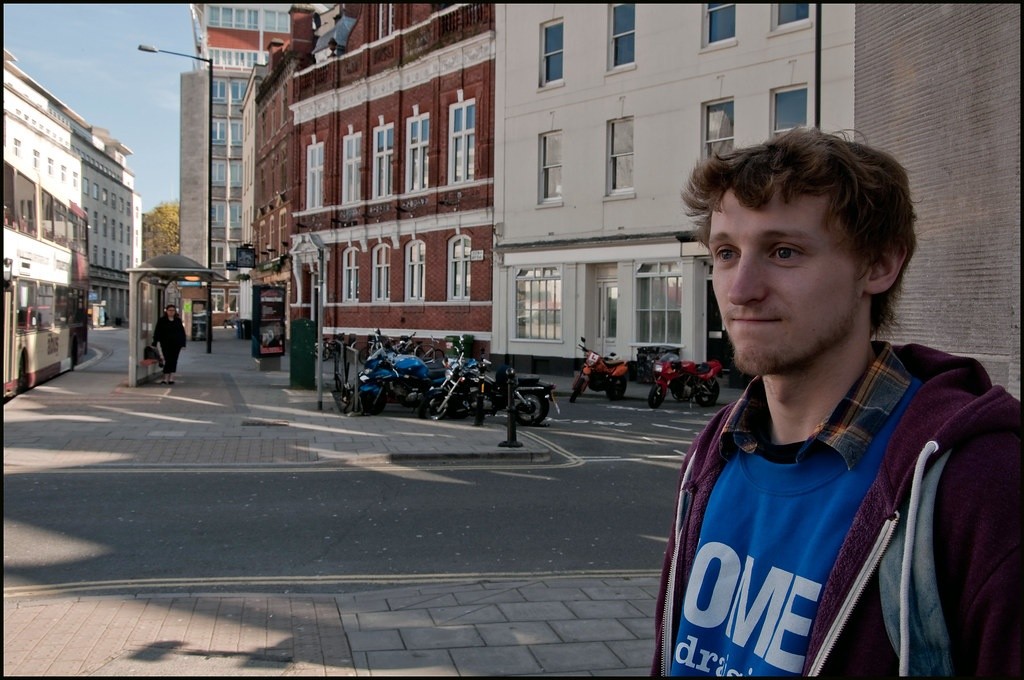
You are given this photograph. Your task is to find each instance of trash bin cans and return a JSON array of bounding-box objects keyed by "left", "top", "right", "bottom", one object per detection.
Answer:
[
  {"left": 636, "top": 346, "right": 680, "bottom": 383},
  {"left": 444, "top": 335, "right": 474, "bottom": 359},
  {"left": 236, "top": 319, "right": 252, "bottom": 340}
]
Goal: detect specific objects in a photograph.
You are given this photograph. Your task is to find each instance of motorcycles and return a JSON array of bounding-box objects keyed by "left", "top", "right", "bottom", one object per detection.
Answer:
[
  {"left": 354, "top": 327, "right": 560, "bottom": 428},
  {"left": 647, "top": 352, "right": 723, "bottom": 409},
  {"left": 569, "top": 336, "right": 630, "bottom": 404}
]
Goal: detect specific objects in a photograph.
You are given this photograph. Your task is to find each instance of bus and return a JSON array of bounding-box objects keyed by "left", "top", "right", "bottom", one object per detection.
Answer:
[{"left": 3, "top": 150, "right": 94, "bottom": 405}]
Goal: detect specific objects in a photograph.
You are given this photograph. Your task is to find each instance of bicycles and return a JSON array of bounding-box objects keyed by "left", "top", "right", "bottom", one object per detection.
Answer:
[{"left": 313, "top": 333, "right": 345, "bottom": 360}]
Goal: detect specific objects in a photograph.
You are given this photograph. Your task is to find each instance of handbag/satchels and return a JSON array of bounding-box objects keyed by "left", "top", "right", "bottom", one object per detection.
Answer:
[{"left": 144, "top": 345, "right": 163, "bottom": 367}]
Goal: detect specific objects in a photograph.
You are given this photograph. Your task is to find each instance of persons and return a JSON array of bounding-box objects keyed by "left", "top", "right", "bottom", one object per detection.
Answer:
[
  {"left": 152, "top": 304, "right": 186, "bottom": 385},
  {"left": 652, "top": 128, "right": 1024, "bottom": 680}
]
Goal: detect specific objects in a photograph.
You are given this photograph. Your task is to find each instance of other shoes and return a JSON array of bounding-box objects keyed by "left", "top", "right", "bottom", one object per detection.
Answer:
[
  {"left": 169, "top": 381, "right": 175, "bottom": 385},
  {"left": 161, "top": 381, "right": 167, "bottom": 385}
]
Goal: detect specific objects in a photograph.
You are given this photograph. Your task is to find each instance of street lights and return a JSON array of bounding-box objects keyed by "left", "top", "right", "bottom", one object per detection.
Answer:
[{"left": 137, "top": 43, "right": 213, "bottom": 354}]
